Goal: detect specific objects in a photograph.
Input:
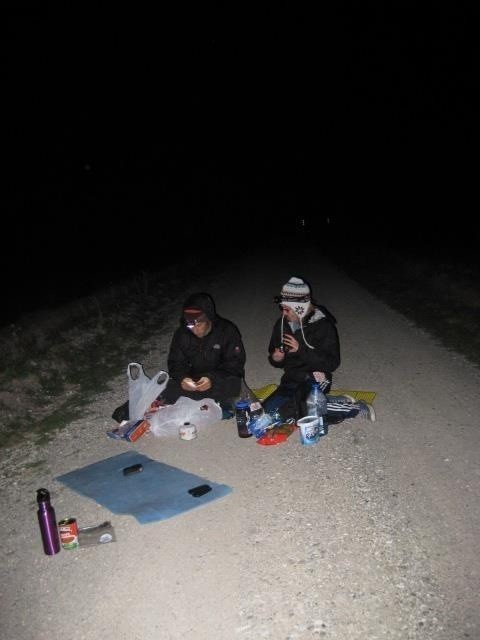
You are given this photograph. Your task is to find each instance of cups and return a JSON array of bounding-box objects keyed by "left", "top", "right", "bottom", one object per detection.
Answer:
[{"left": 296, "top": 416, "right": 320, "bottom": 445}]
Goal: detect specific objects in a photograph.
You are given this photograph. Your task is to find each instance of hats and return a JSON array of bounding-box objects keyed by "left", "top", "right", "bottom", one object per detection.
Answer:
[
  {"left": 277, "top": 275, "right": 313, "bottom": 320},
  {"left": 182, "top": 305, "right": 204, "bottom": 321}
]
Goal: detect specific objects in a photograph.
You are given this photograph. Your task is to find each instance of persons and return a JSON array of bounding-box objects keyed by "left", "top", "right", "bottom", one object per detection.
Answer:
[
  {"left": 166, "top": 291, "right": 247, "bottom": 407},
  {"left": 261, "top": 272, "right": 342, "bottom": 421}
]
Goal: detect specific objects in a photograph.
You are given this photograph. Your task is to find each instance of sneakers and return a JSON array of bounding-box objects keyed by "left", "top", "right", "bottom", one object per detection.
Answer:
[
  {"left": 353, "top": 398, "right": 378, "bottom": 426},
  {"left": 326, "top": 393, "right": 356, "bottom": 404}
]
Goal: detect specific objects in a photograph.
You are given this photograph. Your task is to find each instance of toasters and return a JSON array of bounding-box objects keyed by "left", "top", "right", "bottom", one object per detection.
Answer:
[{"left": 236, "top": 400, "right": 254, "bottom": 438}]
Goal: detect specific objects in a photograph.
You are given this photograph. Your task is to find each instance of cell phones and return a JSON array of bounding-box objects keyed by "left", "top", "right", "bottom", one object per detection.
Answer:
[
  {"left": 122, "top": 464, "right": 144, "bottom": 475},
  {"left": 186, "top": 484, "right": 211, "bottom": 498}
]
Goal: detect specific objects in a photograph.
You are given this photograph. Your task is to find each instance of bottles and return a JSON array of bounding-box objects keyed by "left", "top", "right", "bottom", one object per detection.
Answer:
[
  {"left": 179, "top": 421, "right": 198, "bottom": 440},
  {"left": 35, "top": 488, "right": 61, "bottom": 553},
  {"left": 306, "top": 384, "right": 329, "bottom": 437}
]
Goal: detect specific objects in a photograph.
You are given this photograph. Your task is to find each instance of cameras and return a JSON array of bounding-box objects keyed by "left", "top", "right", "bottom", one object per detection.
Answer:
[{"left": 280, "top": 344, "right": 289, "bottom": 353}]
